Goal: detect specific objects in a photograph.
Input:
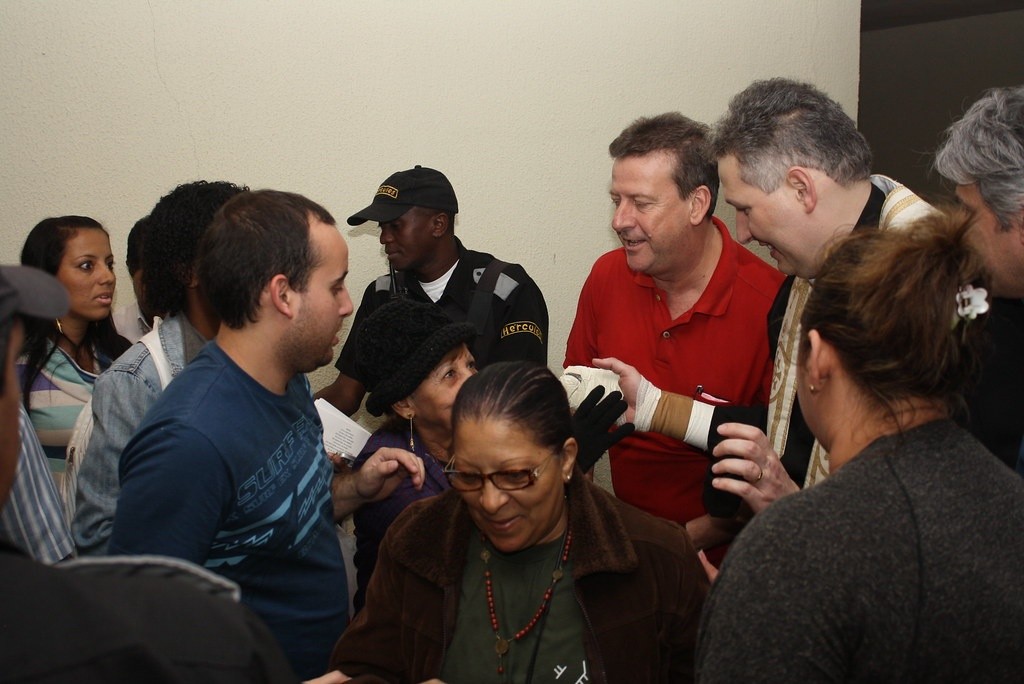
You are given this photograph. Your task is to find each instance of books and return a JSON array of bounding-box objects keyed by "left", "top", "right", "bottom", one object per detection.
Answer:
[{"left": 314, "top": 397, "right": 372, "bottom": 474}]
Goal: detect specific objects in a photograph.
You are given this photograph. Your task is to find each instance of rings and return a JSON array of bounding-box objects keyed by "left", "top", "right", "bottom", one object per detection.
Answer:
[{"left": 753, "top": 468, "right": 763, "bottom": 484}]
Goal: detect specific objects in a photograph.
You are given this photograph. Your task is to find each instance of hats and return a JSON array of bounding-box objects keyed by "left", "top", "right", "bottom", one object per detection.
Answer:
[
  {"left": 346, "top": 165, "right": 459, "bottom": 226},
  {"left": 356, "top": 299, "right": 478, "bottom": 418},
  {"left": 0, "top": 262, "right": 69, "bottom": 325}
]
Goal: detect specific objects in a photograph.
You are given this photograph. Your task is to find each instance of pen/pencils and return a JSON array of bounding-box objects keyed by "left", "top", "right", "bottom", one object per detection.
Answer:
[{"left": 693, "top": 384, "right": 704, "bottom": 401}]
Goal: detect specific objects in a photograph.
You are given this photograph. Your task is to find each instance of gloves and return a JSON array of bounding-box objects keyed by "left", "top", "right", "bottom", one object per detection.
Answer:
[{"left": 569, "top": 385, "right": 636, "bottom": 475}]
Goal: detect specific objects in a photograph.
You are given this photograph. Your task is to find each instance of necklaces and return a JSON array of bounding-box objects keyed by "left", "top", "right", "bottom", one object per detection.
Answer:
[{"left": 480, "top": 510, "right": 572, "bottom": 674}]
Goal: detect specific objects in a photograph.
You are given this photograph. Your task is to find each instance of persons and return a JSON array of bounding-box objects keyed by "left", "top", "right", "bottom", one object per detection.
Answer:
[
  {"left": 310, "top": 165, "right": 549, "bottom": 418},
  {"left": 325, "top": 360, "right": 713, "bottom": 684},
  {"left": 932, "top": 81, "right": 1023, "bottom": 479},
  {"left": 562, "top": 111, "right": 788, "bottom": 598},
  {"left": 102, "top": 193, "right": 425, "bottom": 684},
  {"left": 0, "top": 180, "right": 253, "bottom": 569},
  {"left": 0, "top": 264, "right": 297, "bottom": 684},
  {"left": 692, "top": 206, "right": 1024, "bottom": 684},
  {"left": 556, "top": 77, "right": 988, "bottom": 530},
  {"left": 352, "top": 297, "right": 636, "bottom": 622}
]
